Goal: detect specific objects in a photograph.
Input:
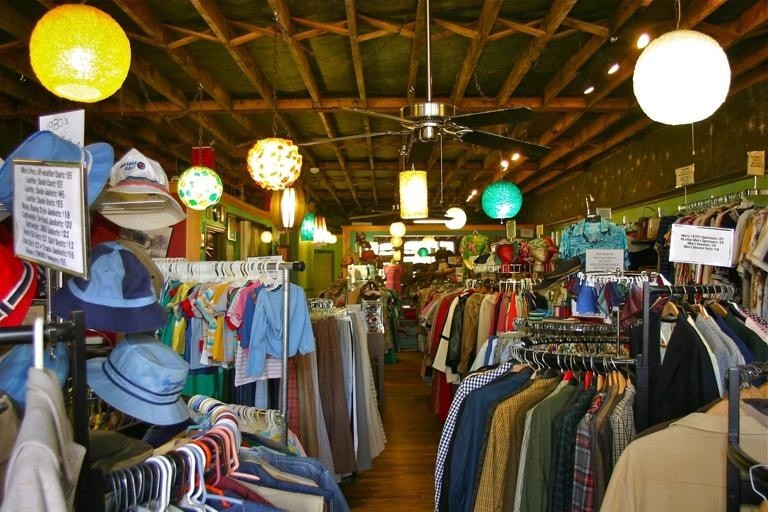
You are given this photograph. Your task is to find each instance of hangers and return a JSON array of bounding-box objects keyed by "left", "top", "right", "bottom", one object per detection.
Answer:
[
  {"left": 33, "top": 316, "right": 45, "bottom": 370},
  {"left": 151, "top": 257, "right": 287, "bottom": 291},
  {"left": 87, "top": 389, "right": 282, "bottom": 512},
  {"left": 463, "top": 190, "right": 768, "bottom": 418},
  {"left": 307, "top": 297, "right": 334, "bottom": 320}
]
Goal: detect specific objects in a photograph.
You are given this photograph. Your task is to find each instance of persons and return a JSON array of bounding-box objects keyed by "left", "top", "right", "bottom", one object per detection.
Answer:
[{"left": 528, "top": 239, "right": 549, "bottom": 274}]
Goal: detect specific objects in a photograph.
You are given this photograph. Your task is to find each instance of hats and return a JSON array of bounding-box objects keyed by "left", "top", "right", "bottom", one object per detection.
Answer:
[
  {"left": 87, "top": 333, "right": 191, "bottom": 425},
  {"left": 0, "top": 131, "right": 115, "bottom": 218},
  {"left": 2, "top": 342, "right": 70, "bottom": 419},
  {"left": 51, "top": 240, "right": 167, "bottom": 334},
  {"left": 1, "top": 224, "right": 38, "bottom": 327},
  {"left": 91, "top": 148, "right": 185, "bottom": 230},
  {"left": 89, "top": 210, "right": 121, "bottom": 248}
]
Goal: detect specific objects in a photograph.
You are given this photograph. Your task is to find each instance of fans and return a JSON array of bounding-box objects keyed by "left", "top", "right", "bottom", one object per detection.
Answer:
[
  {"left": 349, "top": 203, "right": 453, "bottom": 222},
  {"left": 299, "top": 0, "right": 551, "bottom": 165}
]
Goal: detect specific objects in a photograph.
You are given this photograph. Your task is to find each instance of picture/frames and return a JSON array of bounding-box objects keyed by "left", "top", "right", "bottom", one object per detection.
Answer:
[{"left": 227, "top": 213, "right": 237, "bottom": 241}]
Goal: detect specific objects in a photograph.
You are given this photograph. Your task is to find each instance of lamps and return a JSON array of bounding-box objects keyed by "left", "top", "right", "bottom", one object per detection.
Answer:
[
  {"left": 399, "top": 164, "right": 428, "bottom": 220},
  {"left": 177, "top": 88, "right": 224, "bottom": 211},
  {"left": 269, "top": 185, "right": 306, "bottom": 235},
  {"left": 29, "top": 4, "right": 131, "bottom": 105},
  {"left": 632, "top": 0, "right": 732, "bottom": 125},
  {"left": 482, "top": 128, "right": 523, "bottom": 224},
  {"left": 444, "top": 206, "right": 467, "bottom": 230},
  {"left": 246, "top": 21, "right": 303, "bottom": 191},
  {"left": 390, "top": 220, "right": 406, "bottom": 237}
]
